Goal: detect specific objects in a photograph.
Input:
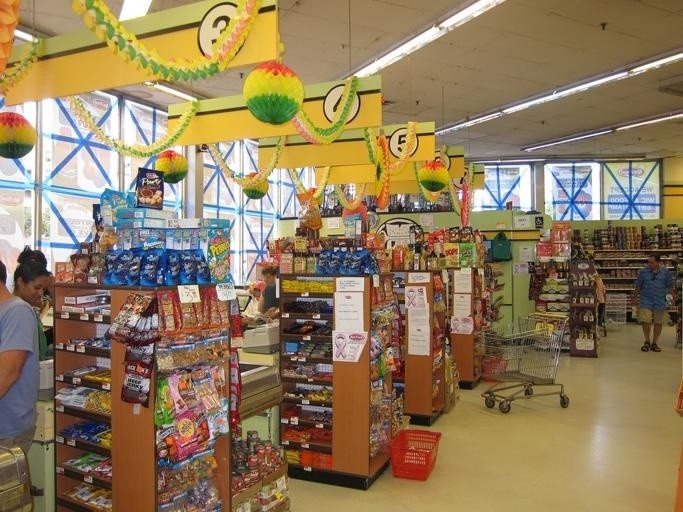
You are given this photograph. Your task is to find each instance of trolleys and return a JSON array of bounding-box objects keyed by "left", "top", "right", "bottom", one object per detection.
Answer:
[
  {"left": 603, "top": 294, "right": 627, "bottom": 331},
  {"left": 673, "top": 296, "right": 681, "bottom": 347},
  {"left": 475, "top": 313, "right": 569, "bottom": 414}
]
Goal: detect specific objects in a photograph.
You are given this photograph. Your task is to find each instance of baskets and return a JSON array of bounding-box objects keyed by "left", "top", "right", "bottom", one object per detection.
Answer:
[{"left": 387, "top": 429, "right": 442, "bottom": 481}]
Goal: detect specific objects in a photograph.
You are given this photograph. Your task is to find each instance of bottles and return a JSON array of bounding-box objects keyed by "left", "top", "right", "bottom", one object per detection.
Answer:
[
  {"left": 542, "top": 263, "right": 547, "bottom": 278},
  {"left": 557, "top": 264, "right": 569, "bottom": 278},
  {"left": 426, "top": 251, "right": 446, "bottom": 271},
  {"left": 413, "top": 246, "right": 421, "bottom": 271}
]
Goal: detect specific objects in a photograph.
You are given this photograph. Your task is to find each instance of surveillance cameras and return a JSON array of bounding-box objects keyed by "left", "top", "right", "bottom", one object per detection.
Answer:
[{"left": 199, "top": 144, "right": 208, "bottom": 152}]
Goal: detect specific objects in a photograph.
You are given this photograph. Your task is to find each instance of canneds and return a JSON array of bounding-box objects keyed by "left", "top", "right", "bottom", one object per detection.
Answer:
[
  {"left": 427, "top": 255, "right": 438, "bottom": 271},
  {"left": 280, "top": 228, "right": 319, "bottom": 275},
  {"left": 231, "top": 427, "right": 283, "bottom": 495}
]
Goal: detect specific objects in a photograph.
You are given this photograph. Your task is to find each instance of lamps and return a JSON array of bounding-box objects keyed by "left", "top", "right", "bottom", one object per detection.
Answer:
[
  {"left": 521, "top": 105, "right": 683, "bottom": 155},
  {"left": 341, "top": 0, "right": 511, "bottom": 85},
  {"left": 429, "top": 44, "right": 683, "bottom": 140},
  {"left": 11, "top": 21, "right": 220, "bottom": 122}
]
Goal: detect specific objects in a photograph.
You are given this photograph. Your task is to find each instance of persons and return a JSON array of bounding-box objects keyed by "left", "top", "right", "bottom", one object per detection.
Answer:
[
  {"left": 0, "top": 246, "right": 55, "bottom": 455},
  {"left": 630, "top": 253, "right": 674, "bottom": 352},
  {"left": 257, "top": 265, "right": 281, "bottom": 314},
  {"left": 597, "top": 280, "right": 606, "bottom": 327}
]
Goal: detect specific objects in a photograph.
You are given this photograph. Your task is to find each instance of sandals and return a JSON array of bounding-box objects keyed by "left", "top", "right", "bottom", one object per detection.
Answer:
[
  {"left": 651, "top": 344, "right": 661, "bottom": 351},
  {"left": 641, "top": 342, "right": 650, "bottom": 351}
]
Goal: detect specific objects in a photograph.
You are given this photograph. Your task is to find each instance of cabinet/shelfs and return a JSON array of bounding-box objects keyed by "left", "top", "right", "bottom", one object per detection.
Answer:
[
  {"left": 268, "top": 266, "right": 410, "bottom": 491},
  {"left": 386, "top": 268, "right": 447, "bottom": 428},
  {"left": 440, "top": 266, "right": 487, "bottom": 390},
  {"left": 235, "top": 439, "right": 291, "bottom": 512},
  {"left": 526, "top": 240, "right": 682, "bottom": 360},
  {"left": 50, "top": 249, "right": 233, "bottom": 510}
]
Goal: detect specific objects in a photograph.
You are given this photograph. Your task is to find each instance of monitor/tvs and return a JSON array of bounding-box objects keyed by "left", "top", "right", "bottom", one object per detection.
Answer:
[{"left": 237, "top": 295, "right": 252, "bottom": 311}]
